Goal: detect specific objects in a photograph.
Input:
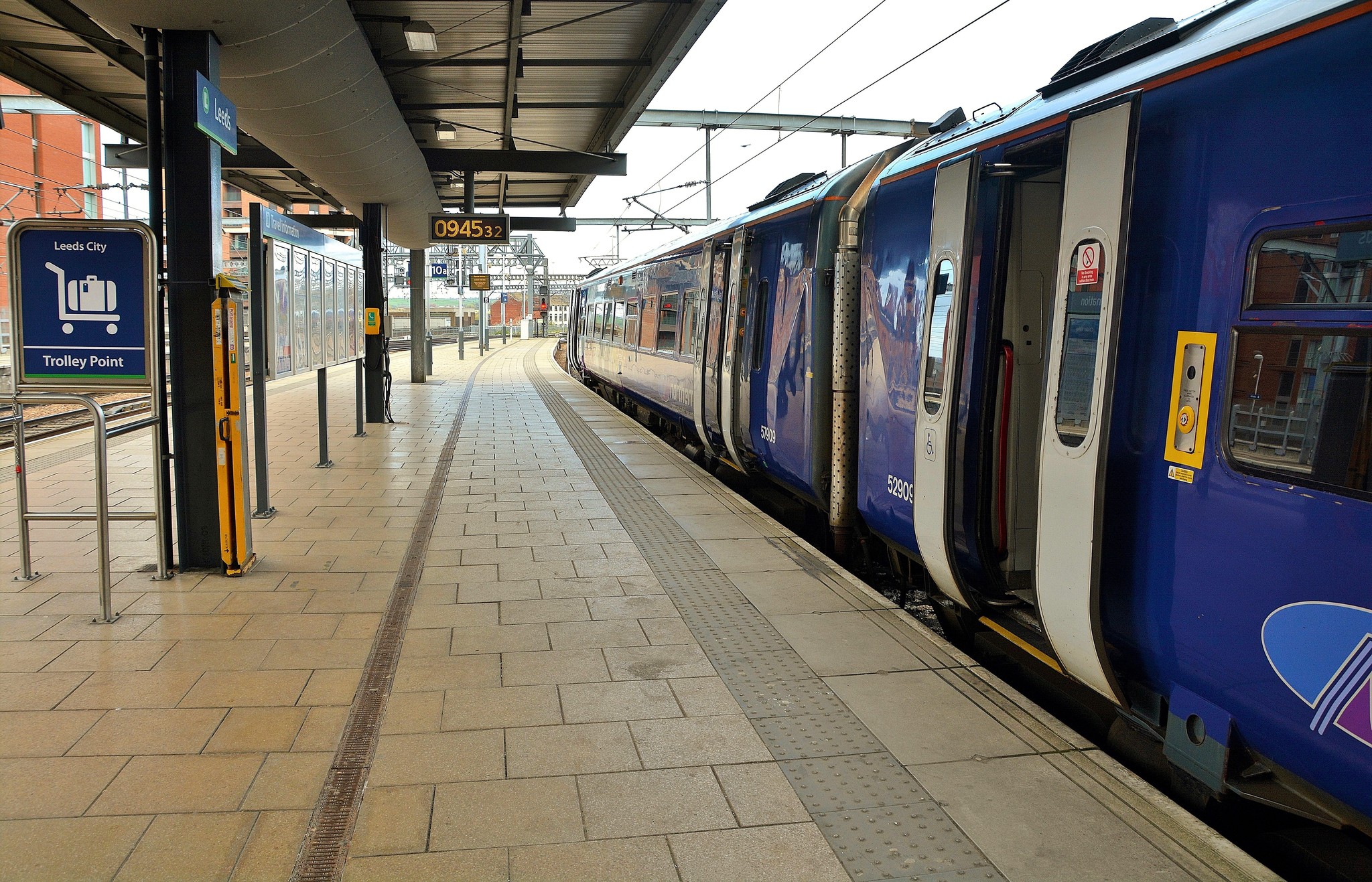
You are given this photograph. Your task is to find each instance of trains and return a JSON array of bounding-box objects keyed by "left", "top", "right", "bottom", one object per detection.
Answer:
[{"left": 565, "top": 1, "right": 1372, "bottom": 833}]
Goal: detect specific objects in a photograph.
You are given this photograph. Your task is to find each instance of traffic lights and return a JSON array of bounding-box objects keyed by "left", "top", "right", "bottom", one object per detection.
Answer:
[
  {"left": 540, "top": 296, "right": 548, "bottom": 309},
  {"left": 406, "top": 272, "right": 411, "bottom": 285}
]
